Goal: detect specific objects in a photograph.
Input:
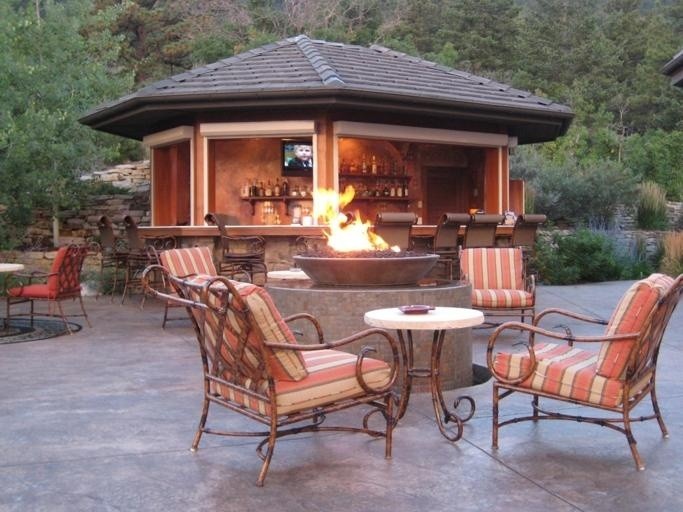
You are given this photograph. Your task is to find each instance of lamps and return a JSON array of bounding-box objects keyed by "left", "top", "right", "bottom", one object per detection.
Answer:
[{"left": 508, "top": 135, "right": 518, "bottom": 156}]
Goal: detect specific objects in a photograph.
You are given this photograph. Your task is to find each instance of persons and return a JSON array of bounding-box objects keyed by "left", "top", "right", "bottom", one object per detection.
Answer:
[{"left": 287, "top": 144, "right": 313, "bottom": 166}]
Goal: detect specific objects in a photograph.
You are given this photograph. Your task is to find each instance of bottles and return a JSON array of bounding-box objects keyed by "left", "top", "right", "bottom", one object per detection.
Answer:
[
  {"left": 341, "top": 153, "right": 398, "bottom": 176},
  {"left": 240, "top": 177, "right": 289, "bottom": 196},
  {"left": 375, "top": 183, "right": 408, "bottom": 198}
]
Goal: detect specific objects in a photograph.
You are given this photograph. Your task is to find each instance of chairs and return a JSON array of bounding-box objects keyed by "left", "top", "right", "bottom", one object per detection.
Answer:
[
  {"left": 3, "top": 242, "right": 92, "bottom": 335},
  {"left": 487, "top": 274, "right": 683, "bottom": 472},
  {"left": 459, "top": 243, "right": 536, "bottom": 334},
  {"left": 143, "top": 267, "right": 399, "bottom": 485},
  {"left": 97, "top": 211, "right": 268, "bottom": 328},
  {"left": 370, "top": 210, "right": 547, "bottom": 281}
]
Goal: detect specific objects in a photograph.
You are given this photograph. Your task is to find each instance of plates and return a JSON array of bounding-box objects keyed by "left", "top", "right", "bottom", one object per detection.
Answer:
[{"left": 398, "top": 304, "right": 435, "bottom": 314}]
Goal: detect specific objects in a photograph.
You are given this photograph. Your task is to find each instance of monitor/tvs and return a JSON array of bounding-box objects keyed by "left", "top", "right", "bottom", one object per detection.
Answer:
[{"left": 282, "top": 139, "right": 313, "bottom": 177}]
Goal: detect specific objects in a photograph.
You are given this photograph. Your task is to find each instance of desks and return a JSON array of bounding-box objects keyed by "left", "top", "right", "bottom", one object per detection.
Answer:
[
  {"left": 0, "top": 262, "right": 25, "bottom": 325},
  {"left": 362, "top": 304, "right": 486, "bottom": 442}
]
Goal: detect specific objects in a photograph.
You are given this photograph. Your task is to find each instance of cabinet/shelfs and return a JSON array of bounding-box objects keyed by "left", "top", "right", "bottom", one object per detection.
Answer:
[{"left": 340, "top": 172, "right": 412, "bottom": 201}]
